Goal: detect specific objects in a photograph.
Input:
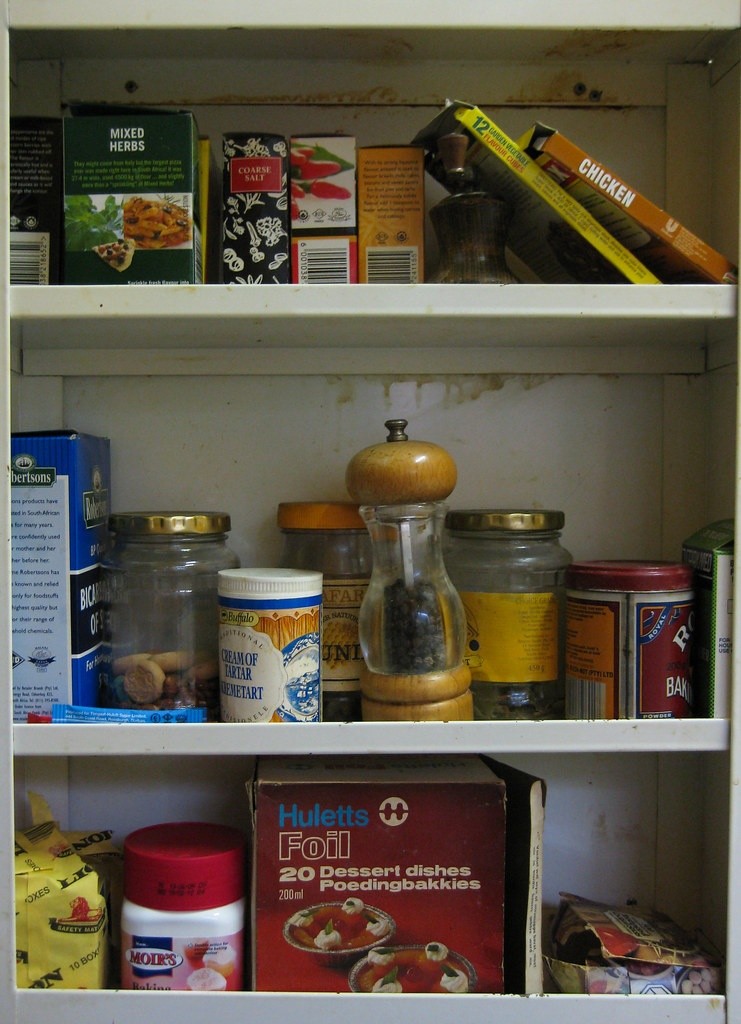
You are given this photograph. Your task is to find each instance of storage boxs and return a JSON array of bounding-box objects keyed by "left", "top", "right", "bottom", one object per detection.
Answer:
[
  {"left": 61, "top": 104, "right": 206, "bottom": 283},
  {"left": 13, "top": 421, "right": 116, "bottom": 720},
  {"left": 17, "top": 804, "right": 117, "bottom": 995},
  {"left": 681, "top": 516, "right": 735, "bottom": 719},
  {"left": 409, "top": 98, "right": 661, "bottom": 285},
  {"left": 289, "top": 133, "right": 354, "bottom": 279},
  {"left": 246, "top": 762, "right": 518, "bottom": 988},
  {"left": 9, "top": 113, "right": 58, "bottom": 283},
  {"left": 198, "top": 133, "right": 223, "bottom": 284},
  {"left": 358, "top": 142, "right": 425, "bottom": 284},
  {"left": 517, "top": 120, "right": 739, "bottom": 285},
  {"left": 226, "top": 129, "right": 289, "bottom": 284}
]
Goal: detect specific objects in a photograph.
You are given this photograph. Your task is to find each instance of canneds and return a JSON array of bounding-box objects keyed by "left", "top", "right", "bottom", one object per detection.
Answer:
[
  {"left": 99, "top": 508, "right": 244, "bottom": 713},
  {"left": 278, "top": 499, "right": 371, "bottom": 720},
  {"left": 438, "top": 507, "right": 571, "bottom": 719},
  {"left": 565, "top": 560, "right": 695, "bottom": 720},
  {"left": 218, "top": 567, "right": 326, "bottom": 724},
  {"left": 112, "top": 823, "right": 245, "bottom": 992}
]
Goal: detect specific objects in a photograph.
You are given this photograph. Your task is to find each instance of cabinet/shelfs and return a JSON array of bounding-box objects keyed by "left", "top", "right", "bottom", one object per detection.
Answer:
[{"left": 0, "top": 0, "right": 741, "bottom": 1024}]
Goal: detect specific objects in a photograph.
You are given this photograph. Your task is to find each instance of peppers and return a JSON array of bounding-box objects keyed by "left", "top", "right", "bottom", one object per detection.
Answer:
[{"left": 288, "top": 146, "right": 352, "bottom": 222}]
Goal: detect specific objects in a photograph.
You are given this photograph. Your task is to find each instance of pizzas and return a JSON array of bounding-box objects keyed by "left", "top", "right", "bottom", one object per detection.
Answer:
[{"left": 93, "top": 238, "right": 137, "bottom": 272}]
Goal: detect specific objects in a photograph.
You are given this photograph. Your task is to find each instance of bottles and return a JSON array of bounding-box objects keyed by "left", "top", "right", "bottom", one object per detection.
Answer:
[
  {"left": 99, "top": 500, "right": 243, "bottom": 721},
  {"left": 428, "top": 133, "right": 523, "bottom": 284},
  {"left": 355, "top": 501, "right": 475, "bottom": 721}
]
